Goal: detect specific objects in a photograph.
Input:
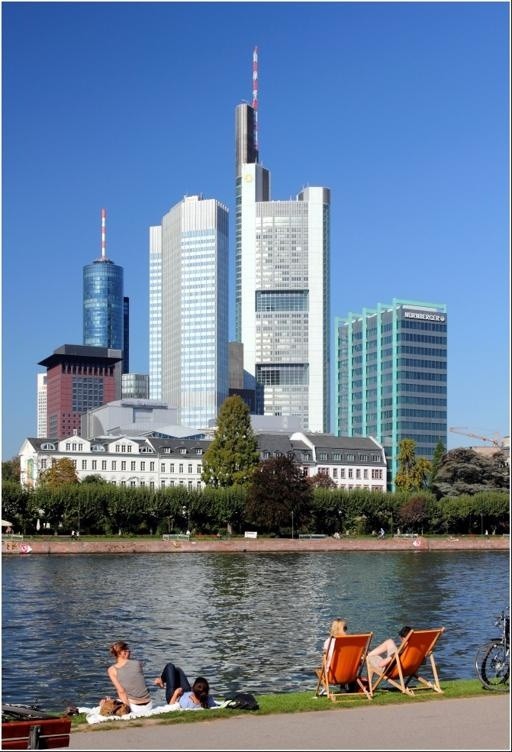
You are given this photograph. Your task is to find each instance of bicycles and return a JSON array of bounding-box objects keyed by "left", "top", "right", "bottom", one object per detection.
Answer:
[{"left": 469, "top": 603, "right": 511, "bottom": 694}]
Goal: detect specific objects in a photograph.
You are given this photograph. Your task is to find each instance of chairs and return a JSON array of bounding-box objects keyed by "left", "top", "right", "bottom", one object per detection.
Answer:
[{"left": 314, "top": 626, "right": 446, "bottom": 702}]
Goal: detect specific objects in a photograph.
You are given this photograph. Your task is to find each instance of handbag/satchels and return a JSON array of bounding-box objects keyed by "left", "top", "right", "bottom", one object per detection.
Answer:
[
  {"left": 100, "top": 700, "right": 131, "bottom": 717},
  {"left": 224, "top": 693, "right": 260, "bottom": 710}
]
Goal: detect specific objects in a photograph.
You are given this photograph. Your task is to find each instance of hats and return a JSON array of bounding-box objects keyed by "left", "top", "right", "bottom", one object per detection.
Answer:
[{"left": 397, "top": 626, "right": 411, "bottom": 637}]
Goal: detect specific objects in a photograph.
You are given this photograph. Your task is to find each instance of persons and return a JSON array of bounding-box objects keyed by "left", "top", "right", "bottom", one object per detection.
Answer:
[
  {"left": 76, "top": 530, "right": 80, "bottom": 541},
  {"left": 98, "top": 641, "right": 154, "bottom": 715},
  {"left": 378, "top": 527, "right": 385, "bottom": 540},
  {"left": 360, "top": 625, "right": 415, "bottom": 684},
  {"left": 153, "top": 663, "right": 216, "bottom": 710},
  {"left": 70, "top": 529, "right": 75, "bottom": 540},
  {"left": 321, "top": 618, "right": 348, "bottom": 680}
]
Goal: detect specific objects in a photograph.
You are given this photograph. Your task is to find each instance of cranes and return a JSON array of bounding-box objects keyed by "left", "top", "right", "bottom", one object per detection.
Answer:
[{"left": 445, "top": 424, "right": 502, "bottom": 447}]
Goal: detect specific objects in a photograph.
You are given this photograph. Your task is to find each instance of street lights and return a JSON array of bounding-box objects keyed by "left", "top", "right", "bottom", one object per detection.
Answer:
[
  {"left": 337, "top": 508, "right": 344, "bottom": 534},
  {"left": 291, "top": 511, "right": 294, "bottom": 539},
  {"left": 479, "top": 513, "right": 484, "bottom": 535},
  {"left": 182, "top": 505, "right": 189, "bottom": 533}
]
